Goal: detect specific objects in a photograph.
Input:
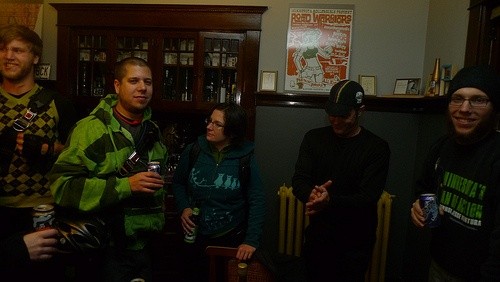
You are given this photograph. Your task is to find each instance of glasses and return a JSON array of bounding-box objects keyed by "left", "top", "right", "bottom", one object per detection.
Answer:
[
  {"left": 207, "top": 118, "right": 225, "bottom": 127},
  {"left": 447, "top": 93, "right": 492, "bottom": 107}
]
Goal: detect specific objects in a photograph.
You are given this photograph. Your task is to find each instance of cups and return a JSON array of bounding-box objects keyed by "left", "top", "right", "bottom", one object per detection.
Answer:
[{"left": 227, "top": 53, "right": 238, "bottom": 67}]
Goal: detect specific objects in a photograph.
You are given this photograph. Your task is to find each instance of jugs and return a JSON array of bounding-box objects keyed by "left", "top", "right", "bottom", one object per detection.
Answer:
[{"left": 208, "top": 43, "right": 229, "bottom": 67}]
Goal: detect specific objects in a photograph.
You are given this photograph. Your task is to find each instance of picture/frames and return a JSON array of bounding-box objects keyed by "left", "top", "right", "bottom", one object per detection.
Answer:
[
  {"left": 393, "top": 78, "right": 408, "bottom": 95},
  {"left": 284, "top": 3, "right": 356, "bottom": 94},
  {"left": 407, "top": 77, "right": 422, "bottom": 95},
  {"left": 259, "top": 70, "right": 279, "bottom": 91},
  {"left": 359, "top": 75, "right": 377, "bottom": 95}
]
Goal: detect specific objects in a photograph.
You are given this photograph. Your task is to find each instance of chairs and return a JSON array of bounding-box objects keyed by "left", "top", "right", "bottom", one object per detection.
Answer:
[{"left": 204, "top": 246, "right": 275, "bottom": 282}]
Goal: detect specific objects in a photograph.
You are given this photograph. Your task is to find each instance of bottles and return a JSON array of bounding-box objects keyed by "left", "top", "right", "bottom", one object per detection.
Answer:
[
  {"left": 184, "top": 208, "right": 199, "bottom": 244},
  {"left": 163, "top": 68, "right": 192, "bottom": 103},
  {"left": 204, "top": 68, "right": 237, "bottom": 104},
  {"left": 237, "top": 263, "right": 247, "bottom": 282},
  {"left": 78, "top": 35, "right": 110, "bottom": 97}
]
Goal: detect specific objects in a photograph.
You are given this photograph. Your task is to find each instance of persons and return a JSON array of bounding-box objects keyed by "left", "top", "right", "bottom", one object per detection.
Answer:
[
  {"left": 290, "top": 80, "right": 389, "bottom": 282},
  {"left": 0, "top": 24, "right": 272, "bottom": 282},
  {"left": 400, "top": 67, "right": 500, "bottom": 282}
]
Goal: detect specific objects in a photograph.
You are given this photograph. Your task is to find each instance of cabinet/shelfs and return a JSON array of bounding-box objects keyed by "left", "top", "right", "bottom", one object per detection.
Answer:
[{"left": 48, "top": 2, "right": 268, "bottom": 152}]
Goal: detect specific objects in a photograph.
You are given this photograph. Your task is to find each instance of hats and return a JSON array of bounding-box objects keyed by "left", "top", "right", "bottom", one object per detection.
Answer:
[
  {"left": 447, "top": 63, "right": 500, "bottom": 110},
  {"left": 326, "top": 79, "right": 366, "bottom": 117}
]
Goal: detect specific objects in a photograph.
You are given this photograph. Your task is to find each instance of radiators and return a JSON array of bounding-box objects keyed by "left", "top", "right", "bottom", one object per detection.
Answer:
[{"left": 278, "top": 186, "right": 391, "bottom": 282}]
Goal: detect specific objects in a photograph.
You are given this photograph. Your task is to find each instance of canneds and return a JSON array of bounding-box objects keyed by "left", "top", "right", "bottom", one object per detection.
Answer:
[
  {"left": 418, "top": 193, "right": 439, "bottom": 228},
  {"left": 147, "top": 162, "right": 160, "bottom": 175},
  {"left": 33, "top": 204, "right": 56, "bottom": 232}
]
